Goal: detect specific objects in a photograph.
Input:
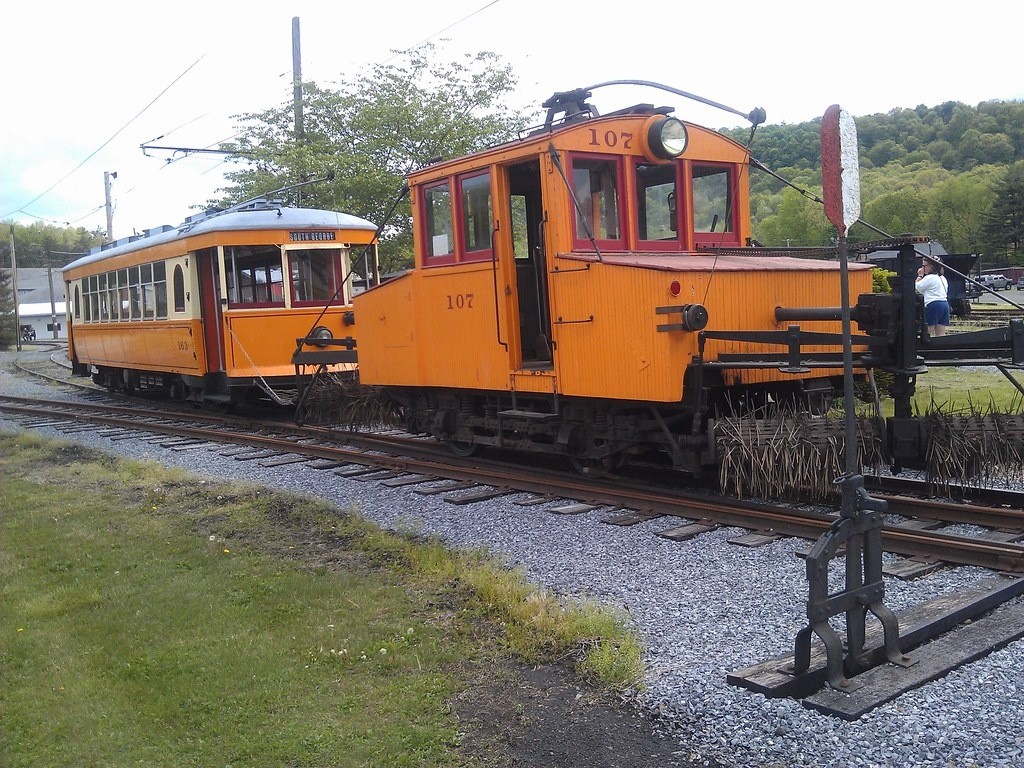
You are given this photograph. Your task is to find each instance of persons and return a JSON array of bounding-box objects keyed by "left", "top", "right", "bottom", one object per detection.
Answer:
[
  {"left": 915, "top": 256, "right": 949, "bottom": 337},
  {"left": 23, "top": 327, "right": 37, "bottom": 341}
]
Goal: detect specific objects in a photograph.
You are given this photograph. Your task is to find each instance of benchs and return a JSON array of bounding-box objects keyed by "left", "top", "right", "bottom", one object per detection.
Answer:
[
  {"left": 966, "top": 291, "right": 983, "bottom": 302},
  {"left": 25, "top": 330, "right": 37, "bottom": 340}
]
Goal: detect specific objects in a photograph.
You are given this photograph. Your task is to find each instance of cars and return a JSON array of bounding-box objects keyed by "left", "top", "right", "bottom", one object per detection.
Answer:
[{"left": 1017, "top": 276, "right": 1024, "bottom": 291}]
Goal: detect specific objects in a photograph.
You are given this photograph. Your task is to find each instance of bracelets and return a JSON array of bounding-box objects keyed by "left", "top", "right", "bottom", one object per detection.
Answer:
[{"left": 918, "top": 274, "right": 923, "bottom": 279}]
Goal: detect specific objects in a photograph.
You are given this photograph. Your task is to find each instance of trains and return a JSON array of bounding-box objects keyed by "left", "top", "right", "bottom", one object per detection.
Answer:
[{"left": 61, "top": 78, "right": 883, "bottom": 488}]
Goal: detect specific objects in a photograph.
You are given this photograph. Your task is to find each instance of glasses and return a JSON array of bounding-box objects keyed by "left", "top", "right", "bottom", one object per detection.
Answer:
[{"left": 920, "top": 264, "right": 928, "bottom": 268}]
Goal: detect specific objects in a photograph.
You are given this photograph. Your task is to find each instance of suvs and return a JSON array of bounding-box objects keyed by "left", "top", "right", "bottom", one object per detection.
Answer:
[{"left": 974, "top": 274, "right": 1013, "bottom": 293}]
